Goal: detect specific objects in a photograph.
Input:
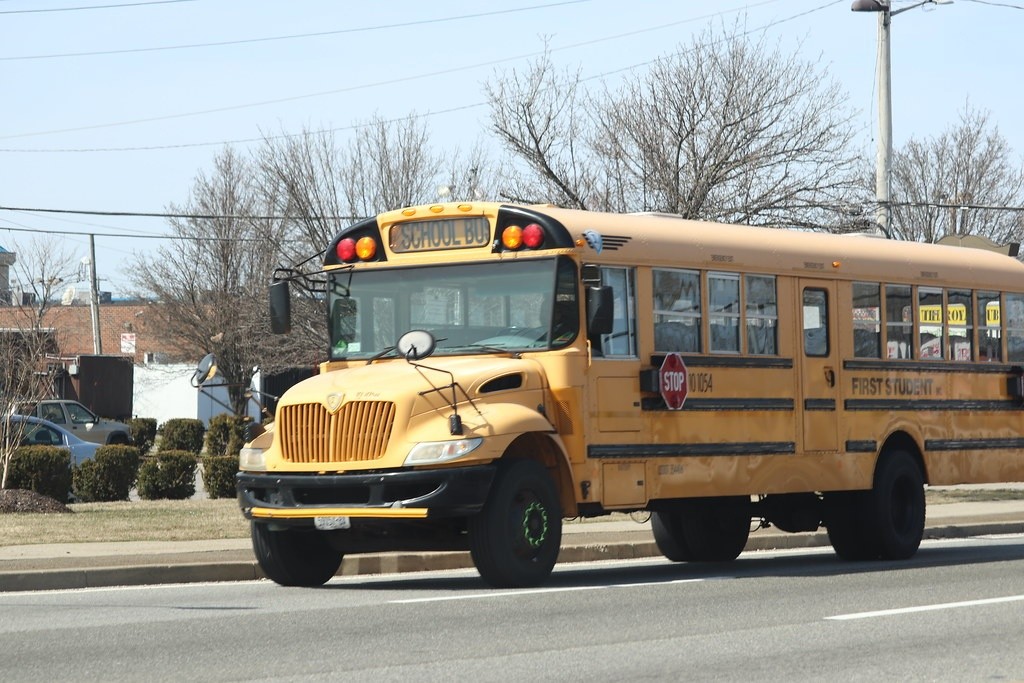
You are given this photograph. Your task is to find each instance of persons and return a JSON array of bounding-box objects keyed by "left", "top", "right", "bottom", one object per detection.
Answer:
[{"left": 539, "top": 296, "right": 577, "bottom": 350}]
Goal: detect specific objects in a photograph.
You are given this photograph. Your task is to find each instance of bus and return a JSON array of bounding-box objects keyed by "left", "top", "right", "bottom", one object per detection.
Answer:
[{"left": 192, "top": 199, "right": 1024, "bottom": 590}]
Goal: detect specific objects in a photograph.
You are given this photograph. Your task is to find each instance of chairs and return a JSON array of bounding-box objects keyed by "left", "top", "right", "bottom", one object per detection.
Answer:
[
  {"left": 35, "top": 429, "right": 55, "bottom": 446},
  {"left": 654, "top": 322, "right": 1024, "bottom": 361}
]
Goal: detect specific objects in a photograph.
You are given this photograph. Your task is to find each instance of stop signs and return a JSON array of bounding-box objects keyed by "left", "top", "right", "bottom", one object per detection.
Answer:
[{"left": 654, "top": 348, "right": 690, "bottom": 412}]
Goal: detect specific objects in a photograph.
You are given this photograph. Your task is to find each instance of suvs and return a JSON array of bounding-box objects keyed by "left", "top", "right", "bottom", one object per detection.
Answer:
[{"left": 5, "top": 400, "right": 135, "bottom": 452}]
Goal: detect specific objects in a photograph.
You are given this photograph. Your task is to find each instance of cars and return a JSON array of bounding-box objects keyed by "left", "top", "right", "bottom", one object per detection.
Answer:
[{"left": 0, "top": 414, "right": 106, "bottom": 498}]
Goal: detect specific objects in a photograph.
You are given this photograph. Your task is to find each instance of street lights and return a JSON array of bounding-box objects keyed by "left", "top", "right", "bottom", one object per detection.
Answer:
[{"left": 850, "top": 1, "right": 898, "bottom": 238}]
